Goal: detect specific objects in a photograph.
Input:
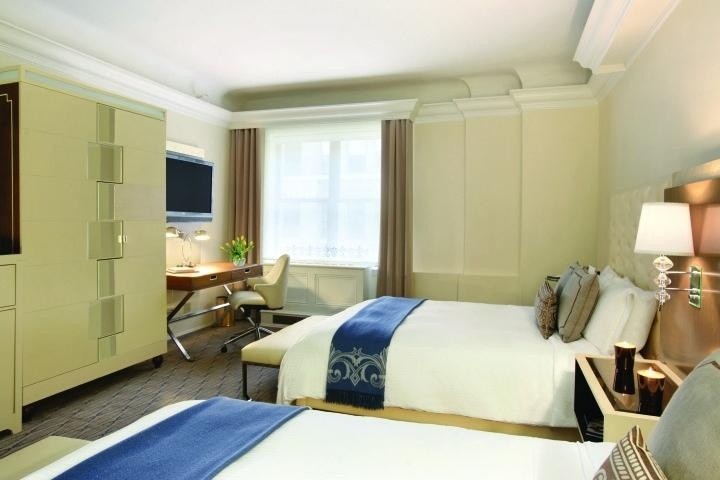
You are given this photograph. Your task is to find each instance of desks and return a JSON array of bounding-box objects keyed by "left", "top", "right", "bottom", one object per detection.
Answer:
[{"left": 166, "top": 261, "right": 264, "bottom": 362}]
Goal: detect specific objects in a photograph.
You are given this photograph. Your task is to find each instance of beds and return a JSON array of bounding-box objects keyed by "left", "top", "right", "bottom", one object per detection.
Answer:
[
  {"left": 274, "top": 296, "right": 644, "bottom": 441},
  {"left": 19, "top": 397, "right": 623, "bottom": 479}
]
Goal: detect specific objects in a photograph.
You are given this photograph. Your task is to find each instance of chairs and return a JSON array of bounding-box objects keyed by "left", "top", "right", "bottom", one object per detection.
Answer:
[{"left": 221, "top": 253, "right": 290, "bottom": 354}]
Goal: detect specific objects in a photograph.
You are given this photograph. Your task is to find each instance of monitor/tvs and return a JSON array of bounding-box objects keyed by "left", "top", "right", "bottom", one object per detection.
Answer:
[{"left": 167, "top": 150, "right": 214, "bottom": 221}]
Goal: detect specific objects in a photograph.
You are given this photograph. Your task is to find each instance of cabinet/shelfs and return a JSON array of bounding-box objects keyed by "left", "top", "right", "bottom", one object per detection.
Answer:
[
  {"left": 0, "top": 264, "right": 16, "bottom": 438},
  {"left": 521, "top": 107, "right": 601, "bottom": 277},
  {"left": 115, "top": 107, "right": 167, "bottom": 356},
  {"left": 457, "top": 276, "right": 547, "bottom": 306},
  {"left": 465, "top": 116, "right": 522, "bottom": 278},
  {"left": 21, "top": 82, "right": 98, "bottom": 388},
  {"left": 414, "top": 276, "right": 458, "bottom": 302},
  {"left": 412, "top": 122, "right": 464, "bottom": 275}
]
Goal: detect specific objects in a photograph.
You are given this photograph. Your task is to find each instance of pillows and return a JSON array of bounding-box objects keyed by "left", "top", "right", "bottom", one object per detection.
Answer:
[
  {"left": 645, "top": 347, "right": 719, "bottom": 480},
  {"left": 556, "top": 264, "right": 601, "bottom": 343},
  {"left": 593, "top": 424, "right": 668, "bottom": 480},
  {"left": 553, "top": 261, "right": 581, "bottom": 307}
]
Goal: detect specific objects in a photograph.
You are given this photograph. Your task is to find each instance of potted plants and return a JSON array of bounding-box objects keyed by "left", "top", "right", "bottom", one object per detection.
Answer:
[{"left": 218, "top": 235, "right": 255, "bottom": 254}]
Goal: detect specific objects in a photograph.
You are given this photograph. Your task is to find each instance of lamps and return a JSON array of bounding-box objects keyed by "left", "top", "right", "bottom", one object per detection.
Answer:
[
  {"left": 176, "top": 229, "right": 210, "bottom": 269},
  {"left": 633, "top": 201, "right": 720, "bottom": 307},
  {"left": 166, "top": 227, "right": 196, "bottom": 268}
]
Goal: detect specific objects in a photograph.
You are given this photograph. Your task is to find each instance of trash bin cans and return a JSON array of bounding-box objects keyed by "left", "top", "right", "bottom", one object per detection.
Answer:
[{"left": 216, "top": 296, "right": 234, "bottom": 327}]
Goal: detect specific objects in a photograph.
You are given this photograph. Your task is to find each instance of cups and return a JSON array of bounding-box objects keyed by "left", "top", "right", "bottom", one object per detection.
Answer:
[
  {"left": 613, "top": 371, "right": 635, "bottom": 394},
  {"left": 614, "top": 343, "right": 636, "bottom": 371}
]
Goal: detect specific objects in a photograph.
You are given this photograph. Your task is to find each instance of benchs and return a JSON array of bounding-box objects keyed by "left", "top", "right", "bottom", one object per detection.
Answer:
[
  {"left": 0, "top": 434, "right": 91, "bottom": 480},
  {"left": 240, "top": 316, "right": 330, "bottom": 402},
  {"left": 533, "top": 280, "right": 559, "bottom": 339}
]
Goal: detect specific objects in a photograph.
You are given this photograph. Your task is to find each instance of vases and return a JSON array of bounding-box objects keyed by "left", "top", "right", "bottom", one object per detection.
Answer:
[{"left": 232, "top": 254, "right": 247, "bottom": 267}]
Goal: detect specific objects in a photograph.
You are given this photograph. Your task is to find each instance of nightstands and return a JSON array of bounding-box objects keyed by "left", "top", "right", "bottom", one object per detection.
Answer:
[{"left": 574, "top": 353, "right": 685, "bottom": 444}]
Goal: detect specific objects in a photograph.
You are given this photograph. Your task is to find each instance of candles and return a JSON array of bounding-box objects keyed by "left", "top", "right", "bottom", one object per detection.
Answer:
[
  {"left": 613, "top": 340, "right": 636, "bottom": 395},
  {"left": 635, "top": 365, "right": 667, "bottom": 417}
]
